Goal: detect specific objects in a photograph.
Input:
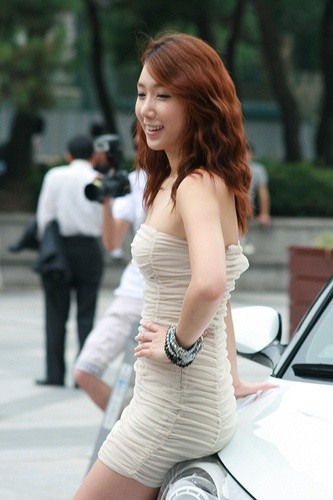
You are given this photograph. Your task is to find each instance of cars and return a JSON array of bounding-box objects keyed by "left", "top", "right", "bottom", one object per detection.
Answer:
[{"left": 157, "top": 272, "right": 333, "bottom": 500}]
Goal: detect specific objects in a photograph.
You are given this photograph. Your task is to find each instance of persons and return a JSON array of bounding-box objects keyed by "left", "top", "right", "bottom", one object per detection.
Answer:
[
  {"left": 34, "top": 136, "right": 105, "bottom": 387},
  {"left": 7, "top": 222, "right": 41, "bottom": 275},
  {"left": 68, "top": 32, "right": 279, "bottom": 500},
  {"left": 247, "top": 142, "right": 271, "bottom": 226},
  {"left": 71, "top": 114, "right": 147, "bottom": 414}
]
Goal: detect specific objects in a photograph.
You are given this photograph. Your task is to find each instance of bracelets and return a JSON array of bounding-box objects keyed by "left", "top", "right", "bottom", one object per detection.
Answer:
[{"left": 164, "top": 326, "right": 203, "bottom": 368}]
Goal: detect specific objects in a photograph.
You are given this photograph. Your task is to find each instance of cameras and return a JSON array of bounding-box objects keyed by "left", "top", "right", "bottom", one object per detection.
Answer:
[{"left": 84, "top": 134, "right": 132, "bottom": 200}]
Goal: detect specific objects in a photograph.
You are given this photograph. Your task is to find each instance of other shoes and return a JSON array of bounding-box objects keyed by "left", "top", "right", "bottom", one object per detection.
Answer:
[{"left": 35, "top": 375, "right": 64, "bottom": 386}]
